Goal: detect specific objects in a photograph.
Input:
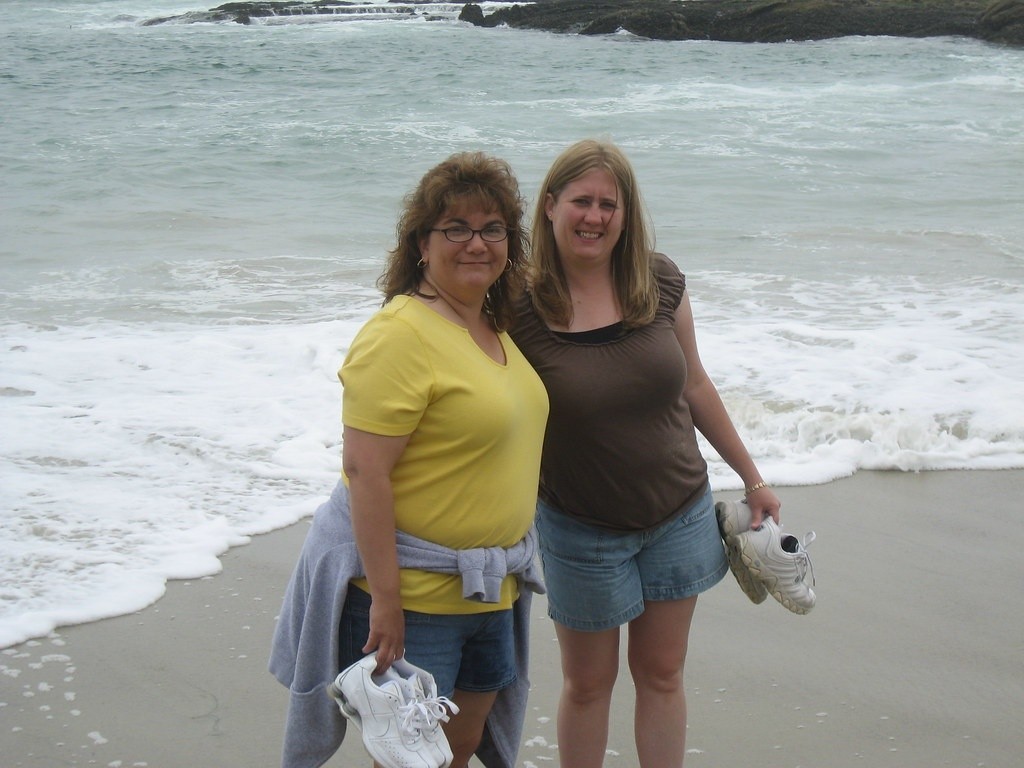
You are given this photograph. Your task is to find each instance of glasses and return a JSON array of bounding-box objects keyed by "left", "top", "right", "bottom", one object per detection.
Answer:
[{"left": 431, "top": 225, "right": 511, "bottom": 244}]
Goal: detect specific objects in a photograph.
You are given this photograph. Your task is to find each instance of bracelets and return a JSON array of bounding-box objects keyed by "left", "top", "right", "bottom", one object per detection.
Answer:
[{"left": 745, "top": 480, "right": 767, "bottom": 495}]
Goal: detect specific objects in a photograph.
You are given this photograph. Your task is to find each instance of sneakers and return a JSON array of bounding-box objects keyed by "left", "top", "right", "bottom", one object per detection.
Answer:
[
  {"left": 715, "top": 497, "right": 770, "bottom": 605},
  {"left": 327, "top": 654, "right": 439, "bottom": 768},
  {"left": 364, "top": 647, "right": 456, "bottom": 768},
  {"left": 733, "top": 514, "right": 817, "bottom": 616}
]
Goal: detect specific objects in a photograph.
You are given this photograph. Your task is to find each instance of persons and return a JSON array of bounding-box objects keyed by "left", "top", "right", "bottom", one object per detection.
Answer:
[
  {"left": 486, "top": 138, "right": 782, "bottom": 768},
  {"left": 301, "top": 149, "right": 550, "bottom": 768}
]
